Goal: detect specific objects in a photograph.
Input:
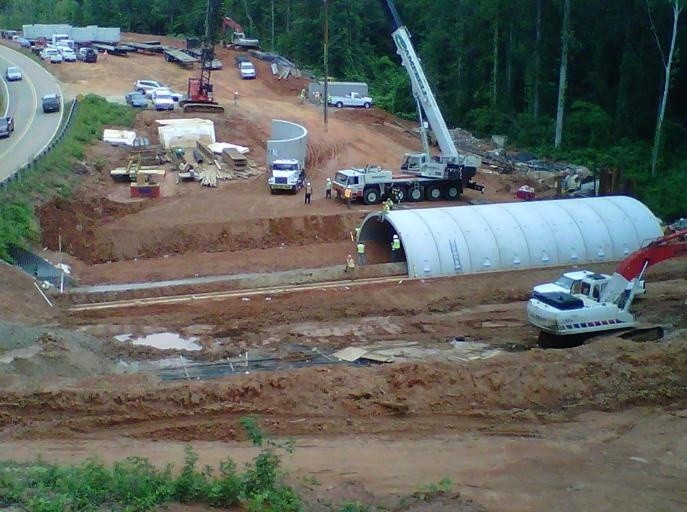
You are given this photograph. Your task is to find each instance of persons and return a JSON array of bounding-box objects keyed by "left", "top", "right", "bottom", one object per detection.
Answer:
[
  {"left": 375, "top": 201, "right": 389, "bottom": 222},
  {"left": 390, "top": 234, "right": 400, "bottom": 262},
  {"left": 355, "top": 223, "right": 359, "bottom": 238},
  {"left": 355, "top": 241, "right": 365, "bottom": 265},
  {"left": 344, "top": 184, "right": 351, "bottom": 209},
  {"left": 343, "top": 254, "right": 355, "bottom": 272},
  {"left": 302, "top": 182, "right": 311, "bottom": 206},
  {"left": 296, "top": 88, "right": 304, "bottom": 103},
  {"left": 233, "top": 91, "right": 241, "bottom": 110},
  {"left": 325, "top": 177, "right": 331, "bottom": 199},
  {"left": 385, "top": 197, "right": 393, "bottom": 210}
]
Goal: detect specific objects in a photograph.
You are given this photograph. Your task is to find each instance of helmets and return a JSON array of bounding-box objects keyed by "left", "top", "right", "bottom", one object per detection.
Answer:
[
  {"left": 327, "top": 178, "right": 330, "bottom": 182},
  {"left": 392, "top": 235, "right": 399, "bottom": 239},
  {"left": 348, "top": 186, "right": 351, "bottom": 189},
  {"left": 307, "top": 182, "right": 310, "bottom": 185}
]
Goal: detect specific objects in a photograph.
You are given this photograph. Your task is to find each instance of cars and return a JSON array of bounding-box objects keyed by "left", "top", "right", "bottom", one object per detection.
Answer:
[
  {"left": 13, "top": 33, "right": 99, "bottom": 64},
  {"left": 5, "top": 66, "right": 23, "bottom": 82},
  {"left": 0, "top": 115, "right": 15, "bottom": 138}
]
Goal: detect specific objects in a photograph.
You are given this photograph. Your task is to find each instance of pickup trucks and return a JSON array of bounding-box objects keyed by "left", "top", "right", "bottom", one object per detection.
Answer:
[{"left": 327, "top": 91, "right": 376, "bottom": 110}]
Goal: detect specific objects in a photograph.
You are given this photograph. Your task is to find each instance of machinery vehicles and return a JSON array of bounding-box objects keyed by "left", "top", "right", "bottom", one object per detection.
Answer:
[
  {"left": 266, "top": 157, "right": 310, "bottom": 195},
  {"left": 179, "top": 1, "right": 226, "bottom": 115},
  {"left": 525, "top": 226, "right": 687, "bottom": 346},
  {"left": 218, "top": 12, "right": 262, "bottom": 53},
  {"left": 328, "top": 0, "right": 488, "bottom": 207}
]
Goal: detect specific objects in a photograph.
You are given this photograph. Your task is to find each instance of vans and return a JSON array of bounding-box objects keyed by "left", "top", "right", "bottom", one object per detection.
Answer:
[{"left": 40, "top": 93, "right": 62, "bottom": 113}]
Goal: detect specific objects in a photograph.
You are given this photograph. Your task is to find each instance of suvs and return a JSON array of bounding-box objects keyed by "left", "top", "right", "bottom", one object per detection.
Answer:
[
  {"left": 237, "top": 60, "right": 257, "bottom": 79},
  {"left": 133, "top": 77, "right": 182, "bottom": 110}
]
